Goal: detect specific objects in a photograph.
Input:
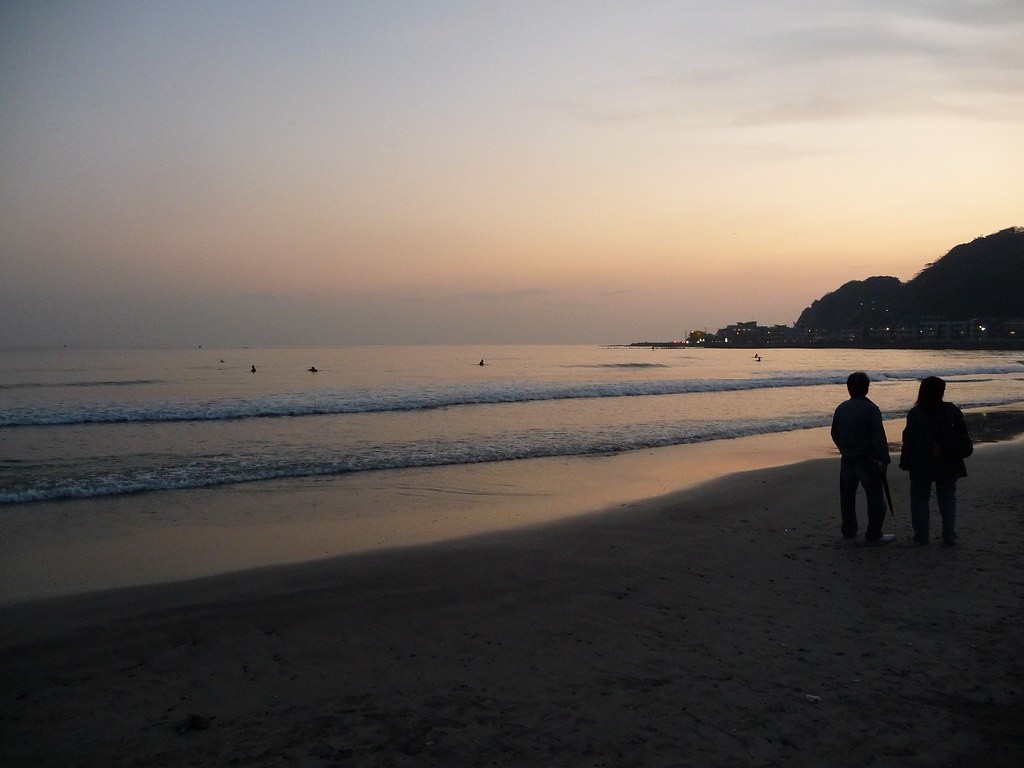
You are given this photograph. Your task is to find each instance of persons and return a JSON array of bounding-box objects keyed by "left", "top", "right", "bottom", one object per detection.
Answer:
[
  {"left": 831, "top": 371, "right": 896, "bottom": 542},
  {"left": 899, "top": 375, "right": 974, "bottom": 546}
]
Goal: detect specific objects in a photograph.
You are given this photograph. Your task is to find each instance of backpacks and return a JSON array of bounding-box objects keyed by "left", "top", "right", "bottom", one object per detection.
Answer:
[{"left": 937, "top": 412, "right": 973, "bottom": 458}]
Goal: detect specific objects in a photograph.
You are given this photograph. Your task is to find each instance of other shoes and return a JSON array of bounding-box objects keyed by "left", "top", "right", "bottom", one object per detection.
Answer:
[{"left": 876, "top": 534, "right": 896, "bottom": 542}]
